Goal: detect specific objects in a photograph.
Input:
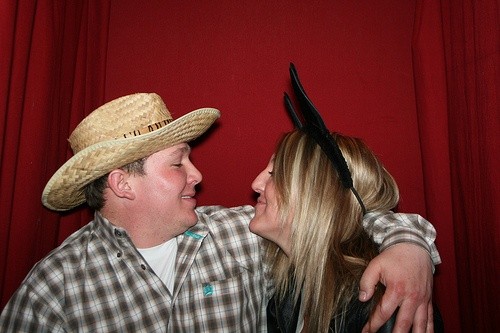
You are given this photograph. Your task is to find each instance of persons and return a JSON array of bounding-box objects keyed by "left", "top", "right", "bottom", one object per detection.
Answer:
[
  {"left": 1, "top": 93, "right": 442, "bottom": 333},
  {"left": 249, "top": 128, "right": 442, "bottom": 333}
]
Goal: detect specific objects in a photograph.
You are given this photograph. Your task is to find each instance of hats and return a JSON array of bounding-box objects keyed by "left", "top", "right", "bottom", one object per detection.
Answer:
[{"left": 42, "top": 92, "right": 221, "bottom": 210}]
